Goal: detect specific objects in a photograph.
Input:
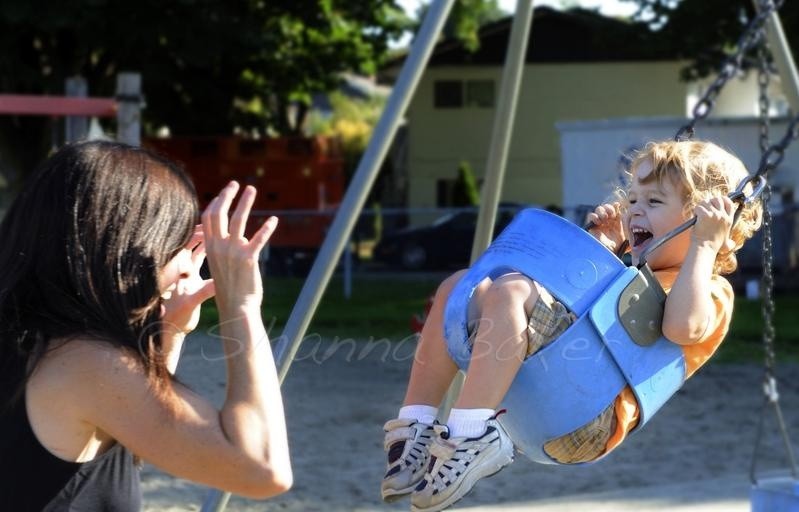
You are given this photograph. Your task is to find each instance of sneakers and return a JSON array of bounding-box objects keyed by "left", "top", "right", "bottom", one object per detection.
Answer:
[
  {"left": 381, "top": 419, "right": 444, "bottom": 502},
  {"left": 409, "top": 417, "right": 514, "bottom": 511}
]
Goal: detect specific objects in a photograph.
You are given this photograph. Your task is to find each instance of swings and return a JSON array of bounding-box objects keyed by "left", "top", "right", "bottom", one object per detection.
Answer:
[
  {"left": 443, "top": 0, "right": 799, "bottom": 467},
  {"left": 749, "top": 48, "right": 799, "bottom": 512}
]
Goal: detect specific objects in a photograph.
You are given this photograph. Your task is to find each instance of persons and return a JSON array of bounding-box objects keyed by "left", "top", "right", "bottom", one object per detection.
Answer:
[
  {"left": 1, "top": 139, "right": 294, "bottom": 511},
  {"left": 382, "top": 137, "right": 763, "bottom": 511}
]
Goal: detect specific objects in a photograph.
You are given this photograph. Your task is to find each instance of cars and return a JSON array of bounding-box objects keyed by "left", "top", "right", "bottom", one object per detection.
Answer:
[
  {"left": 722, "top": 182, "right": 799, "bottom": 295},
  {"left": 380, "top": 202, "right": 554, "bottom": 271}
]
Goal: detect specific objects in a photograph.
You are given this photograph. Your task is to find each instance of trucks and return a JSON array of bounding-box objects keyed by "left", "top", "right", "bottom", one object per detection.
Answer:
[{"left": 138, "top": 134, "right": 345, "bottom": 277}]
